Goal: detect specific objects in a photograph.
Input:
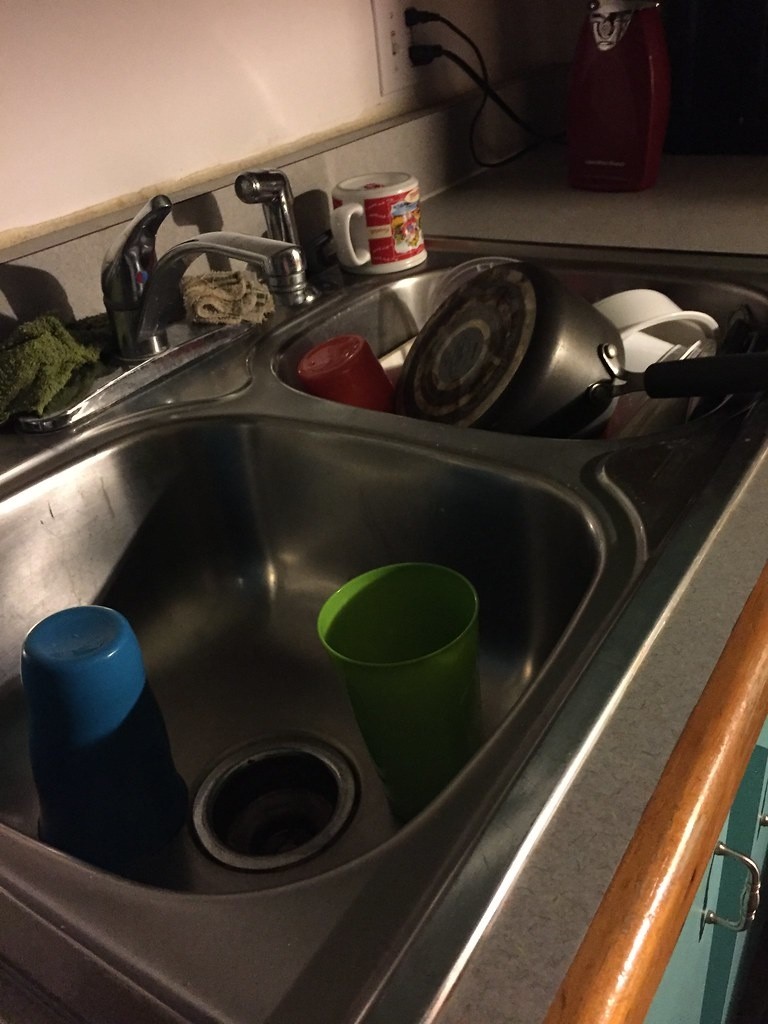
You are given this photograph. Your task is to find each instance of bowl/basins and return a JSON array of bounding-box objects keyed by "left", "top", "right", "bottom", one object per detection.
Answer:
[
  {"left": 591, "top": 287, "right": 722, "bottom": 372},
  {"left": 424, "top": 257, "right": 526, "bottom": 320}
]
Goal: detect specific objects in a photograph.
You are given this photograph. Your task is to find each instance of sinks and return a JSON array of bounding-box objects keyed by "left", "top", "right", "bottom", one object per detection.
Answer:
[
  {"left": 0, "top": 393, "right": 651, "bottom": 1023},
  {"left": 250, "top": 242, "right": 768, "bottom": 465}
]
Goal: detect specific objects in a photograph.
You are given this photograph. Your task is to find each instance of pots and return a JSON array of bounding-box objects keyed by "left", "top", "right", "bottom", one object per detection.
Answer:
[{"left": 402, "top": 263, "right": 768, "bottom": 433}]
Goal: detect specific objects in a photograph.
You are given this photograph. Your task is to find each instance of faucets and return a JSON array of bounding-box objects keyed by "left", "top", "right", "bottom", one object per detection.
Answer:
[{"left": 99, "top": 195, "right": 307, "bottom": 363}]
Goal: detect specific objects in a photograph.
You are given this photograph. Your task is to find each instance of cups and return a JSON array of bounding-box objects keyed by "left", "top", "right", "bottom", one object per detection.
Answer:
[
  {"left": 297, "top": 336, "right": 395, "bottom": 415},
  {"left": 331, "top": 172, "right": 428, "bottom": 274},
  {"left": 316, "top": 564, "right": 479, "bottom": 823},
  {"left": 19, "top": 606, "right": 191, "bottom": 871}
]
